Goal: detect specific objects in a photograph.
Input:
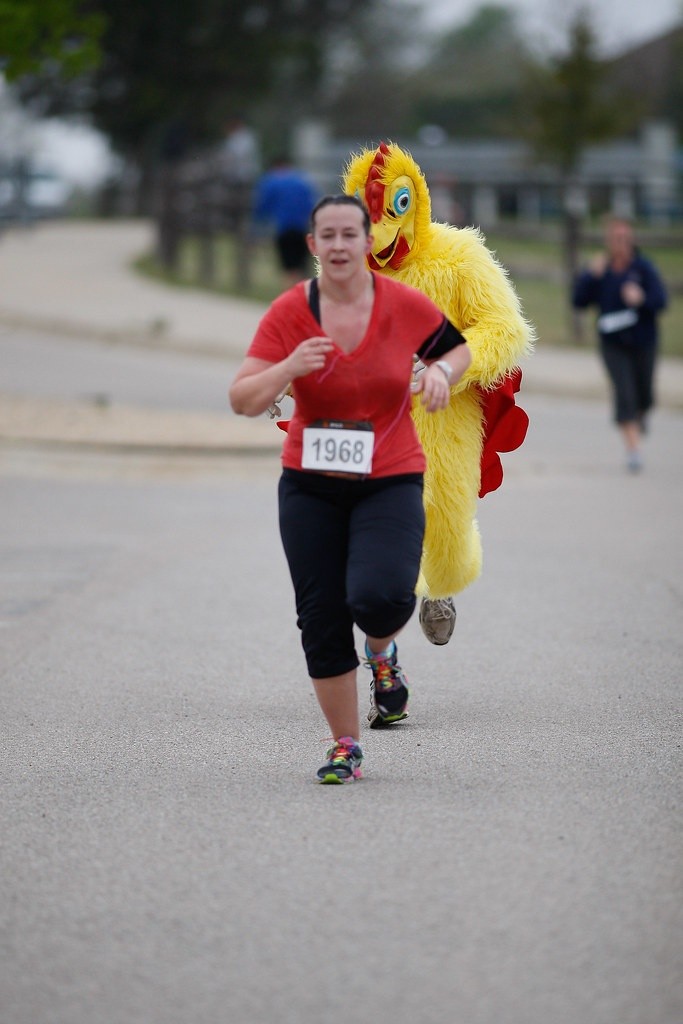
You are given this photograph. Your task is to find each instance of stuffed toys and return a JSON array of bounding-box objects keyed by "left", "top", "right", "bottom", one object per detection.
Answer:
[{"left": 312, "top": 137, "right": 539, "bottom": 733}]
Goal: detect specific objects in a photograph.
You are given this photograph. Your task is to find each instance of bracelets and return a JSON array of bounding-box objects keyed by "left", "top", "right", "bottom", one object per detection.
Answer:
[{"left": 433, "top": 361, "right": 453, "bottom": 383}]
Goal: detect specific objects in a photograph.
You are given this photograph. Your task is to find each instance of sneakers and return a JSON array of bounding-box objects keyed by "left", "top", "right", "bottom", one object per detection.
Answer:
[
  {"left": 362, "top": 638, "right": 408, "bottom": 719},
  {"left": 317, "top": 739, "right": 365, "bottom": 785},
  {"left": 365, "top": 659, "right": 406, "bottom": 728},
  {"left": 420, "top": 594, "right": 458, "bottom": 643}
]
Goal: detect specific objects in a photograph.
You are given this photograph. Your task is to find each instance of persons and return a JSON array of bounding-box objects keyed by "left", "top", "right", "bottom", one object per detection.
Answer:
[
  {"left": 251, "top": 151, "right": 322, "bottom": 294},
  {"left": 227, "top": 192, "right": 474, "bottom": 787},
  {"left": 570, "top": 213, "right": 672, "bottom": 468}
]
